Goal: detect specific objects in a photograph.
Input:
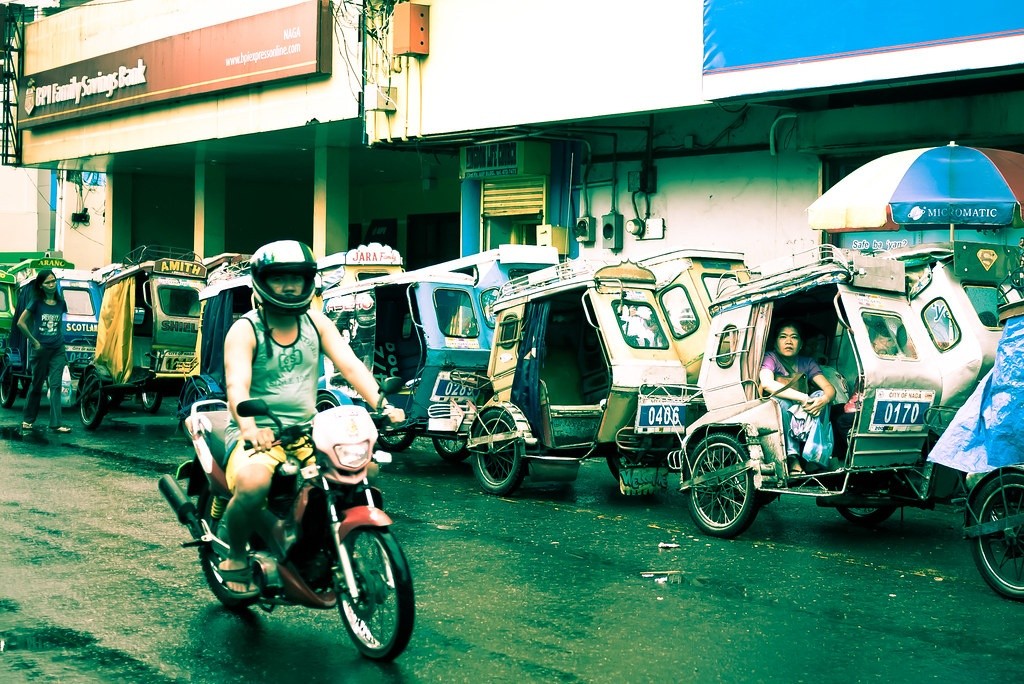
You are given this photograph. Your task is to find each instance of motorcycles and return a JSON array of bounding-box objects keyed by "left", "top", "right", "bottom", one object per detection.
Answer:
[
  {"left": 157, "top": 376, "right": 422, "bottom": 665},
  {"left": 0, "top": 219, "right": 983, "bottom": 534},
  {"left": 926, "top": 288, "right": 1024, "bottom": 601}
]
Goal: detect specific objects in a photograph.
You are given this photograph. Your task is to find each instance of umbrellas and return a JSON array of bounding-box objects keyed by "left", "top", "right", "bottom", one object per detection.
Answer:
[{"left": 808, "top": 141, "right": 1024, "bottom": 240}]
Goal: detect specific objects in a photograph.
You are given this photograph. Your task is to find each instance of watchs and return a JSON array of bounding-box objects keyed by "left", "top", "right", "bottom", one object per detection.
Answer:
[{"left": 383, "top": 403, "right": 395, "bottom": 410}]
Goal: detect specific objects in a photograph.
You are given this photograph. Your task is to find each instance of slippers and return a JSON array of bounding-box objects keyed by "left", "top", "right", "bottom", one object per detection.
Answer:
[
  {"left": 208, "top": 552, "right": 260, "bottom": 599},
  {"left": 789, "top": 469, "right": 806, "bottom": 476}
]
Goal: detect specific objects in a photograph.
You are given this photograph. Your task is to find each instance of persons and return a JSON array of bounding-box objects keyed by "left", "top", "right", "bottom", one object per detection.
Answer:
[
  {"left": 800, "top": 324, "right": 898, "bottom": 469},
  {"left": 217, "top": 239, "right": 405, "bottom": 599},
  {"left": 17, "top": 269, "right": 71, "bottom": 433},
  {"left": 758, "top": 319, "right": 836, "bottom": 475}
]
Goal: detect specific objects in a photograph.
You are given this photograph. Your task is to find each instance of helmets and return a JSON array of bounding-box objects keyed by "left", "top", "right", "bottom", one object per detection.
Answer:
[{"left": 249, "top": 240, "right": 316, "bottom": 317}]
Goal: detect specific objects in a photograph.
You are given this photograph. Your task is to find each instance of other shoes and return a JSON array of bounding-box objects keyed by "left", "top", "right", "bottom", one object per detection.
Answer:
[
  {"left": 22, "top": 421, "right": 33, "bottom": 429},
  {"left": 57, "top": 427, "right": 72, "bottom": 433}
]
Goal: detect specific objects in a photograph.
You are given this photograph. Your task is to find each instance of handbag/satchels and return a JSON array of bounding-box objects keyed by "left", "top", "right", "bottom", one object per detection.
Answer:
[{"left": 802, "top": 402, "right": 834, "bottom": 467}]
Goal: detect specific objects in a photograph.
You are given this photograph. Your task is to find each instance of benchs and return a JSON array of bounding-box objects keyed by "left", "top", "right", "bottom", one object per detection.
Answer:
[
  {"left": 366, "top": 337, "right": 422, "bottom": 410},
  {"left": 131, "top": 331, "right": 154, "bottom": 368},
  {"left": 536, "top": 349, "right": 606, "bottom": 448}
]
